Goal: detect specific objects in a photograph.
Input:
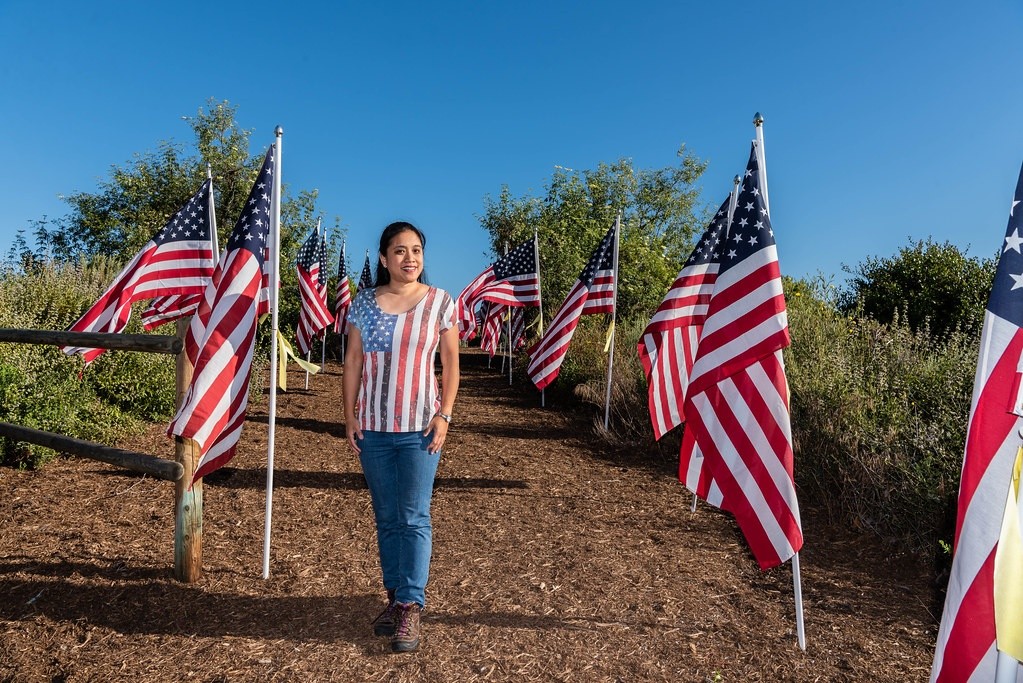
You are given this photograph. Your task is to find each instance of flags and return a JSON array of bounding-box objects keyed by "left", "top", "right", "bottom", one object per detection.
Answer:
[
  {"left": 166, "top": 141, "right": 275, "bottom": 489},
  {"left": 679, "top": 139, "right": 803, "bottom": 571},
  {"left": 141, "top": 293, "right": 203, "bottom": 332},
  {"left": 475, "top": 299, "right": 527, "bottom": 358},
  {"left": 356, "top": 256, "right": 373, "bottom": 293},
  {"left": 297, "top": 226, "right": 335, "bottom": 354},
  {"left": 928, "top": 155, "right": 1023, "bottom": 683},
  {"left": 454, "top": 235, "right": 540, "bottom": 342},
  {"left": 527, "top": 218, "right": 614, "bottom": 392},
  {"left": 59, "top": 176, "right": 215, "bottom": 370},
  {"left": 314, "top": 234, "right": 327, "bottom": 342},
  {"left": 334, "top": 246, "right": 352, "bottom": 335},
  {"left": 637, "top": 194, "right": 734, "bottom": 442}
]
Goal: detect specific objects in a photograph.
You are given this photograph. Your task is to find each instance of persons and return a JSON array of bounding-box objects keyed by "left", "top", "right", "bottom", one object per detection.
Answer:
[{"left": 343, "top": 222, "right": 459, "bottom": 652}]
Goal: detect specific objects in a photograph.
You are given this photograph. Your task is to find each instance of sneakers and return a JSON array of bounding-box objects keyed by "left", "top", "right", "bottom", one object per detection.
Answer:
[
  {"left": 370, "top": 587, "right": 396, "bottom": 636},
  {"left": 391, "top": 601, "right": 420, "bottom": 652}
]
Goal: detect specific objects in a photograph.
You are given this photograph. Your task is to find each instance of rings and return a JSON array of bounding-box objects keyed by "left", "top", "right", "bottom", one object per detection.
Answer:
[{"left": 346, "top": 440, "right": 348, "bottom": 444}]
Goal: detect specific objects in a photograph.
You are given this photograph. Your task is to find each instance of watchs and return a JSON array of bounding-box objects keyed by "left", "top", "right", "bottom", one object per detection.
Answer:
[{"left": 438, "top": 413, "right": 451, "bottom": 422}]
